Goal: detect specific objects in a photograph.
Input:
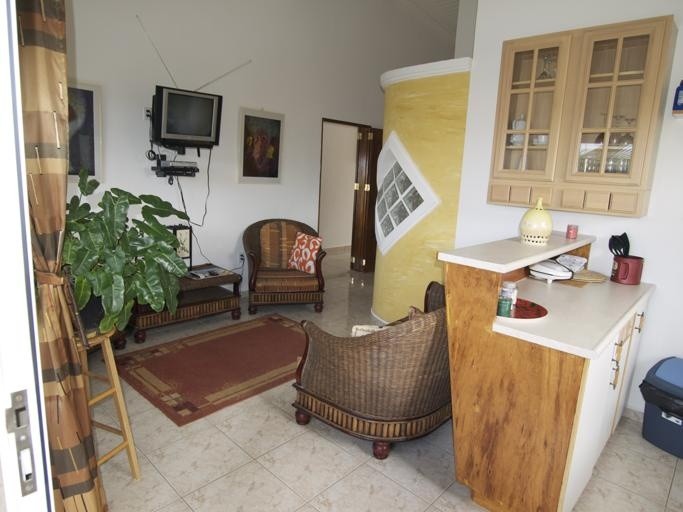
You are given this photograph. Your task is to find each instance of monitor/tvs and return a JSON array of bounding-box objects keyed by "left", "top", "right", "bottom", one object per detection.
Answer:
[{"left": 152, "top": 86, "right": 223, "bottom": 146}]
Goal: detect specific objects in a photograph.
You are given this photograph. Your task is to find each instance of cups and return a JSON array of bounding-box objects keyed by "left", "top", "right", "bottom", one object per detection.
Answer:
[
  {"left": 579, "top": 158, "right": 629, "bottom": 174},
  {"left": 536, "top": 134, "right": 548, "bottom": 146},
  {"left": 566, "top": 224, "right": 578, "bottom": 239},
  {"left": 610, "top": 253, "right": 645, "bottom": 286}
]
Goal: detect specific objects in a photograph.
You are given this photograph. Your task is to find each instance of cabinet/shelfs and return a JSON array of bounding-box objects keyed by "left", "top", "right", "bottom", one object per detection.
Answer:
[
  {"left": 436, "top": 228, "right": 656, "bottom": 511},
  {"left": 486, "top": 13, "right": 678, "bottom": 219},
  {"left": 126, "top": 264, "right": 242, "bottom": 343}
]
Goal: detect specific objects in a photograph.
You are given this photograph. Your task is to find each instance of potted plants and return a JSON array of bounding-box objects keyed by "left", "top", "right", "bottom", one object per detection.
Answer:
[{"left": 61, "top": 167, "right": 200, "bottom": 328}]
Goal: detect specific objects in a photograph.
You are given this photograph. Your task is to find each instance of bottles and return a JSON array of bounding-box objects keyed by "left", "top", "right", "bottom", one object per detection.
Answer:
[{"left": 497, "top": 282, "right": 520, "bottom": 316}]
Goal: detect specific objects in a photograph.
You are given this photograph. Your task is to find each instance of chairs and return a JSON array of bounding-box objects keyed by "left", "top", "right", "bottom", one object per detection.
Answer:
[
  {"left": 243, "top": 218, "right": 327, "bottom": 315},
  {"left": 292, "top": 281, "right": 451, "bottom": 459}
]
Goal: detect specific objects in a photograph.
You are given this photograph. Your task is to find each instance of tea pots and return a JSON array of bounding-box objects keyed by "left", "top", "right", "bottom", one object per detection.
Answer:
[{"left": 510, "top": 111, "right": 529, "bottom": 147}]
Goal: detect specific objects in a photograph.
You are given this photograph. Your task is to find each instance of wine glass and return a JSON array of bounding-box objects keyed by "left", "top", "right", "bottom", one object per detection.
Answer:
[
  {"left": 536, "top": 53, "right": 553, "bottom": 81},
  {"left": 594, "top": 112, "right": 634, "bottom": 145}
]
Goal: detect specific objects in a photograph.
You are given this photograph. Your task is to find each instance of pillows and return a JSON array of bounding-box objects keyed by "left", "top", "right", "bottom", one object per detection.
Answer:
[{"left": 288, "top": 230, "right": 323, "bottom": 274}]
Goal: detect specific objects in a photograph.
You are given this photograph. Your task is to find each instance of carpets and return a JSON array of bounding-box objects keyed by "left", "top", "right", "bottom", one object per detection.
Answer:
[{"left": 100, "top": 312, "right": 306, "bottom": 427}]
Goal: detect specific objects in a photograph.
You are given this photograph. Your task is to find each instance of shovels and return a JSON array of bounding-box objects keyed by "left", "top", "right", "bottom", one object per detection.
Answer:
[
  {"left": 619, "top": 232, "right": 629, "bottom": 256},
  {"left": 609, "top": 237, "right": 625, "bottom": 256}
]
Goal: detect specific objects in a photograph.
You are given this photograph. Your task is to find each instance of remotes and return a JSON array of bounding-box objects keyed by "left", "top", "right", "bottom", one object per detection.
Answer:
[
  {"left": 190, "top": 270, "right": 205, "bottom": 279},
  {"left": 209, "top": 269, "right": 216, "bottom": 276}
]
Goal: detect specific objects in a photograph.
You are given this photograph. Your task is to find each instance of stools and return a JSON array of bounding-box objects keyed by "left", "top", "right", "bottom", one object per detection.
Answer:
[{"left": 72, "top": 327, "right": 141, "bottom": 480}]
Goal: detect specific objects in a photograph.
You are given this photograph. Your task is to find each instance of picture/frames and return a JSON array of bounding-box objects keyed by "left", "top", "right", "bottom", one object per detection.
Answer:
[
  {"left": 66, "top": 86, "right": 102, "bottom": 184},
  {"left": 239, "top": 106, "right": 285, "bottom": 184}
]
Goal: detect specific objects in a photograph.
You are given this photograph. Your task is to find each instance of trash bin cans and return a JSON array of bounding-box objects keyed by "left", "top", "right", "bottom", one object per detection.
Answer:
[{"left": 642, "top": 356, "right": 683, "bottom": 459}]
